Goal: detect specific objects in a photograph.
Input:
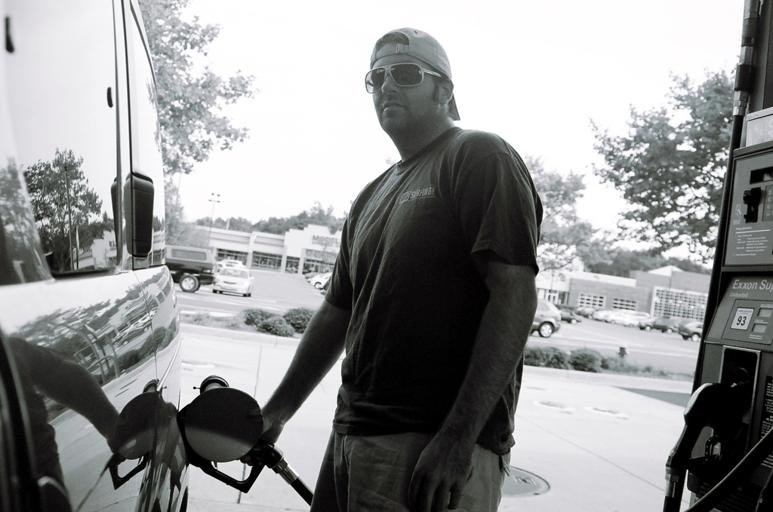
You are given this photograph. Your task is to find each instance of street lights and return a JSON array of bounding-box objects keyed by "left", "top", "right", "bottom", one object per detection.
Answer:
[{"left": 207, "top": 192, "right": 221, "bottom": 243}]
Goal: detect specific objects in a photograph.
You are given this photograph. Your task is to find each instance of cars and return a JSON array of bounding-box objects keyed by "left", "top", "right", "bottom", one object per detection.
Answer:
[
  {"left": 86, "top": 310, "right": 154, "bottom": 377},
  {"left": 1, "top": 0, "right": 272, "bottom": 511},
  {"left": 165, "top": 244, "right": 253, "bottom": 297},
  {"left": 148, "top": 242, "right": 165, "bottom": 274},
  {"left": 529, "top": 297, "right": 704, "bottom": 342},
  {"left": 302, "top": 269, "right": 334, "bottom": 290}
]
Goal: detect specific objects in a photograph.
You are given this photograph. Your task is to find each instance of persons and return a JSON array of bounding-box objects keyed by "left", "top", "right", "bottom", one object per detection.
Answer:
[{"left": 255, "top": 27, "right": 543, "bottom": 512}]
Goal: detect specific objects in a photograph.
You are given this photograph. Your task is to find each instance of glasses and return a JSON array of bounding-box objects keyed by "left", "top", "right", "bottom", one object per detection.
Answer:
[{"left": 365, "top": 62, "right": 444, "bottom": 94}]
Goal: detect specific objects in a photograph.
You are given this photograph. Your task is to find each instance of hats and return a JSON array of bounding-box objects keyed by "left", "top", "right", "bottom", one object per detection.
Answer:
[{"left": 369, "top": 28, "right": 460, "bottom": 120}]
